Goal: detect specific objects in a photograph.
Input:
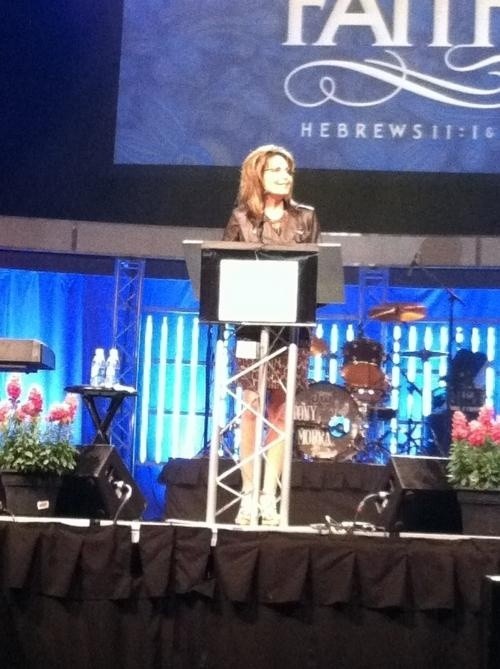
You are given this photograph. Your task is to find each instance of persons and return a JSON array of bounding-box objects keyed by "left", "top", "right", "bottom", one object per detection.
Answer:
[{"left": 217, "top": 143, "right": 325, "bottom": 525}]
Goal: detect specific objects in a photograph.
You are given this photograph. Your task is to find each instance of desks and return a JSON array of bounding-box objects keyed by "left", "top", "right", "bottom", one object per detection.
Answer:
[{"left": 55, "top": 383, "right": 138, "bottom": 515}]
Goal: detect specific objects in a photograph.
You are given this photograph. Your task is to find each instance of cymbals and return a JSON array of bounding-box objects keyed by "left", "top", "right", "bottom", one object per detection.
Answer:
[
  {"left": 367, "top": 302, "right": 427, "bottom": 323},
  {"left": 310, "top": 336, "right": 329, "bottom": 356},
  {"left": 398, "top": 348, "right": 448, "bottom": 360}
]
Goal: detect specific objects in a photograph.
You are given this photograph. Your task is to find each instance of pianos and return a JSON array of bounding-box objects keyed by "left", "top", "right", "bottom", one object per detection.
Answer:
[{"left": 0, "top": 339, "right": 56, "bottom": 374}]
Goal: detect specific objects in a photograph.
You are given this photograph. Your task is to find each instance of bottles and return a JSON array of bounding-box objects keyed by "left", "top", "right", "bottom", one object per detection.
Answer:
[
  {"left": 90, "top": 346, "right": 105, "bottom": 385},
  {"left": 105, "top": 347, "right": 120, "bottom": 388}
]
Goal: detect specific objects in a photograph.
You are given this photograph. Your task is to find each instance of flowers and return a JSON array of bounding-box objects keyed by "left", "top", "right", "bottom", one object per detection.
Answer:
[
  {"left": 0, "top": 376, "right": 78, "bottom": 475},
  {"left": 447, "top": 404, "right": 498, "bottom": 491}
]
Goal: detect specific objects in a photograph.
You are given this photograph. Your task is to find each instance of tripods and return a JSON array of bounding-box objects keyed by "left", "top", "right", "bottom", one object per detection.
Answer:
[
  {"left": 358, "top": 402, "right": 390, "bottom": 462},
  {"left": 387, "top": 361, "right": 427, "bottom": 454}
]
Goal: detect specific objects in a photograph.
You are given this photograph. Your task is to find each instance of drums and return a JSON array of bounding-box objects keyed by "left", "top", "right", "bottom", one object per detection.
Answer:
[
  {"left": 352, "top": 375, "right": 391, "bottom": 403},
  {"left": 293, "top": 382, "right": 366, "bottom": 462},
  {"left": 341, "top": 339, "right": 385, "bottom": 388}
]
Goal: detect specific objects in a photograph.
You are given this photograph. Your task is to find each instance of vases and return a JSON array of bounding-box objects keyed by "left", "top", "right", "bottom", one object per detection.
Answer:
[
  {"left": 454, "top": 489, "right": 499, "bottom": 536},
  {"left": 2, "top": 475, "right": 64, "bottom": 517}
]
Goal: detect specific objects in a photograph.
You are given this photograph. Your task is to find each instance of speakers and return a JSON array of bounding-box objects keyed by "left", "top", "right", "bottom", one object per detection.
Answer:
[
  {"left": 67, "top": 444, "right": 149, "bottom": 521},
  {"left": 372, "top": 455, "right": 463, "bottom": 534}
]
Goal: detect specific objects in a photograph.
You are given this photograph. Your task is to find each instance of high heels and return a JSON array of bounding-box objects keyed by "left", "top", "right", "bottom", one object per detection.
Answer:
[
  {"left": 233, "top": 488, "right": 259, "bottom": 525},
  {"left": 259, "top": 493, "right": 280, "bottom": 525}
]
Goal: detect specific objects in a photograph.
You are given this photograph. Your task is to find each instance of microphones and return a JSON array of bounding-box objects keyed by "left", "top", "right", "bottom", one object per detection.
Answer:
[
  {"left": 404, "top": 251, "right": 423, "bottom": 278},
  {"left": 257, "top": 190, "right": 271, "bottom": 241}
]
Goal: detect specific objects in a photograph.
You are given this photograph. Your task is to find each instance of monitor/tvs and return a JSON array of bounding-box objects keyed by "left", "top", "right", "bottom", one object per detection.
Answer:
[{"left": 199, "top": 248, "right": 319, "bottom": 326}]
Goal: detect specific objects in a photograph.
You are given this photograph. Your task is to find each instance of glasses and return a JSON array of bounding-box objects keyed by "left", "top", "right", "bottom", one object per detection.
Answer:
[{"left": 265, "top": 167, "right": 293, "bottom": 175}]
